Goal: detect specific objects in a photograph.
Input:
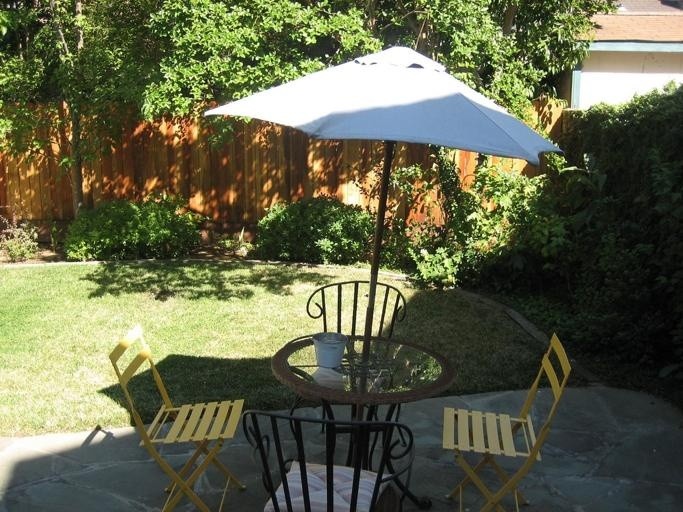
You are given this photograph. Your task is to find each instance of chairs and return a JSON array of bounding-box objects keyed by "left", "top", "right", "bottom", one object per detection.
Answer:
[
  {"left": 108, "top": 323, "right": 246, "bottom": 512},
  {"left": 306, "top": 280, "right": 408, "bottom": 448},
  {"left": 241, "top": 410, "right": 413, "bottom": 512},
  {"left": 441, "top": 332, "right": 572, "bottom": 511}
]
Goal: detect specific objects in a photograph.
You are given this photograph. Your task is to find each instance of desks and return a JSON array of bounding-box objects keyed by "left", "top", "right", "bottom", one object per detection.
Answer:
[{"left": 272, "top": 334, "right": 456, "bottom": 511}]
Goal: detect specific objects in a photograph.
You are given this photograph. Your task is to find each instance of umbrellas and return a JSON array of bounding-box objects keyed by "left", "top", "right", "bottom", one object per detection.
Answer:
[{"left": 204, "top": 47, "right": 566, "bottom": 419}]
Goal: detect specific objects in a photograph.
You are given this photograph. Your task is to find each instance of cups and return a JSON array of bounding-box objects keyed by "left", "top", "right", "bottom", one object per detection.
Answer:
[{"left": 310, "top": 332, "right": 349, "bottom": 368}]
